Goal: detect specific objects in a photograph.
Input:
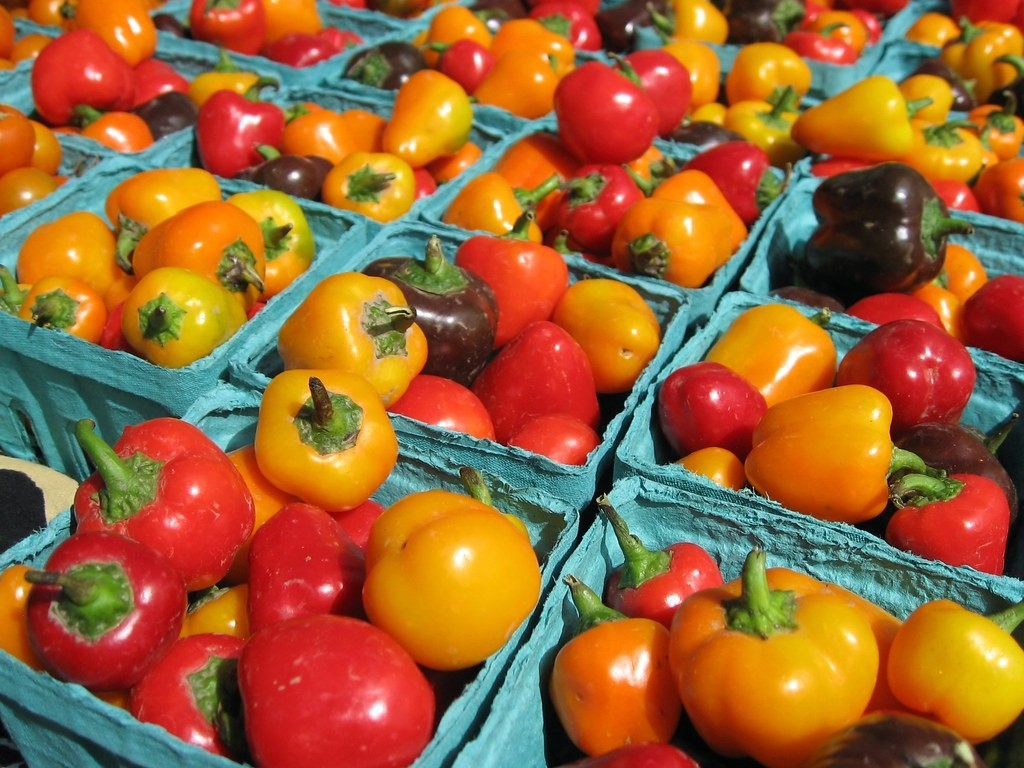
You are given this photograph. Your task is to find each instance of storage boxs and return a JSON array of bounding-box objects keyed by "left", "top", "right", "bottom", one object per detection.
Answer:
[{"left": 0, "top": 0, "right": 1024, "bottom": 768}]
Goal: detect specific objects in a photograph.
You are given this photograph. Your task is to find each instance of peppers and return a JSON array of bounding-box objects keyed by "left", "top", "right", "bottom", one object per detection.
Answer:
[{"left": 0, "top": 0, "right": 1024, "bottom": 768}]
